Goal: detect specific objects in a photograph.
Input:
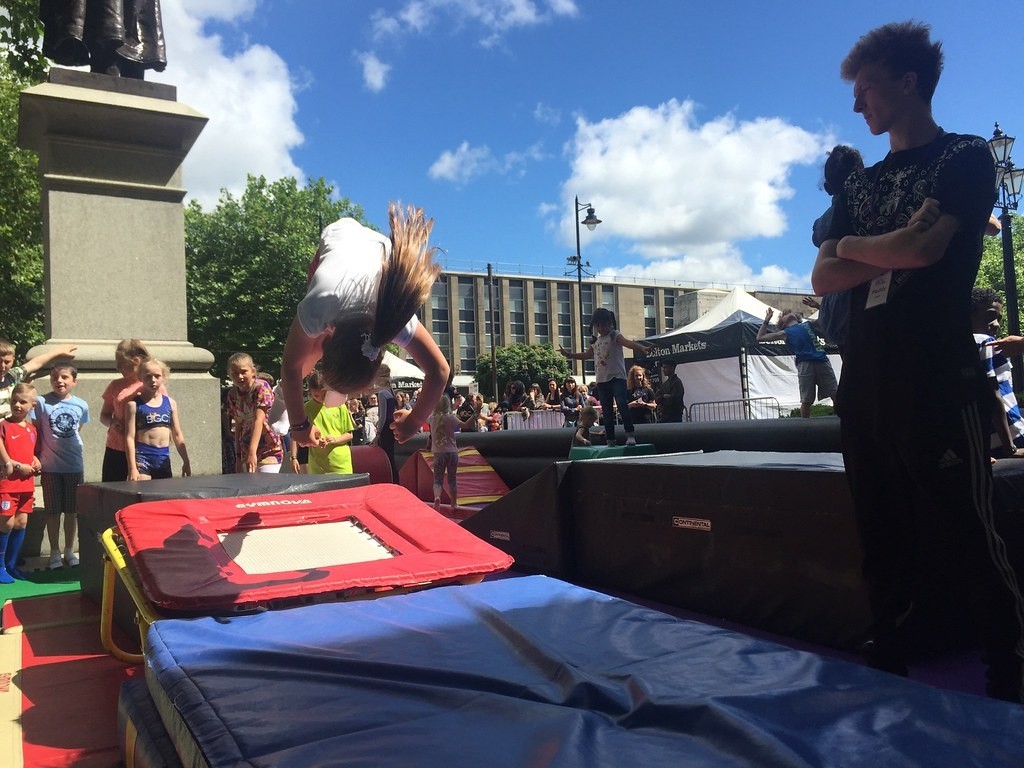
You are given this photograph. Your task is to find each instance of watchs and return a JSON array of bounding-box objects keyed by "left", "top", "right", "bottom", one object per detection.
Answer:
[{"left": 290, "top": 456, "right": 298, "bottom": 461}]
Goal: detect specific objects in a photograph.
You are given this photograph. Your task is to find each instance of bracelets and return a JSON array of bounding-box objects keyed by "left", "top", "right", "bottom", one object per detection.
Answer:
[
  {"left": 15, "top": 463, "right": 21, "bottom": 470},
  {"left": 291, "top": 416, "right": 310, "bottom": 432},
  {"left": 3, "top": 461, "right": 10, "bottom": 468},
  {"left": 764, "top": 321, "right": 768, "bottom": 325}
]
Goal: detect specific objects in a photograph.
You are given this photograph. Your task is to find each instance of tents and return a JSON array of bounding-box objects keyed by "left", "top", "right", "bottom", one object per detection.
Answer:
[
  {"left": 633, "top": 285, "right": 842, "bottom": 422},
  {"left": 366, "top": 351, "right": 425, "bottom": 391}
]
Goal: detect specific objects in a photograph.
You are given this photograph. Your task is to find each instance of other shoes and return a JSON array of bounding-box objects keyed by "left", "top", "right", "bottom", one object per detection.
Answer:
[
  {"left": 604, "top": 440, "right": 616, "bottom": 448},
  {"left": 48, "top": 551, "right": 63, "bottom": 570},
  {"left": 64, "top": 549, "right": 79, "bottom": 567},
  {"left": 626, "top": 436, "right": 636, "bottom": 445}
]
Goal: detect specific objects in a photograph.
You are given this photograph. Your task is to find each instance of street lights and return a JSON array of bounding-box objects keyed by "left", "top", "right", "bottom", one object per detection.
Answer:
[
  {"left": 563, "top": 194, "right": 602, "bottom": 385},
  {"left": 987, "top": 121, "right": 1024, "bottom": 395}
]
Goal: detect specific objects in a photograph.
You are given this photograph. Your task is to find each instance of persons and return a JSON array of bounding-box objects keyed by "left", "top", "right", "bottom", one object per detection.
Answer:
[
  {"left": 451, "top": 377, "right": 603, "bottom": 445},
  {"left": 0, "top": 337, "right": 90, "bottom": 584},
  {"left": 426, "top": 395, "right": 484, "bottom": 513},
  {"left": 812, "top": 22, "right": 1024, "bottom": 703},
  {"left": 228, "top": 353, "right": 309, "bottom": 474},
  {"left": 374, "top": 364, "right": 400, "bottom": 485},
  {"left": 281, "top": 199, "right": 450, "bottom": 447},
  {"left": 657, "top": 358, "right": 685, "bottom": 423},
  {"left": 556, "top": 308, "right": 653, "bottom": 447},
  {"left": 394, "top": 387, "right": 422, "bottom": 434},
  {"left": 124, "top": 359, "right": 191, "bottom": 481},
  {"left": 755, "top": 296, "right": 839, "bottom": 418},
  {"left": 346, "top": 394, "right": 379, "bottom": 446},
  {"left": 39, "top": 0, "right": 168, "bottom": 80},
  {"left": 100, "top": 338, "right": 169, "bottom": 482},
  {"left": 304, "top": 370, "right": 355, "bottom": 473},
  {"left": 626, "top": 365, "right": 657, "bottom": 424}
]
